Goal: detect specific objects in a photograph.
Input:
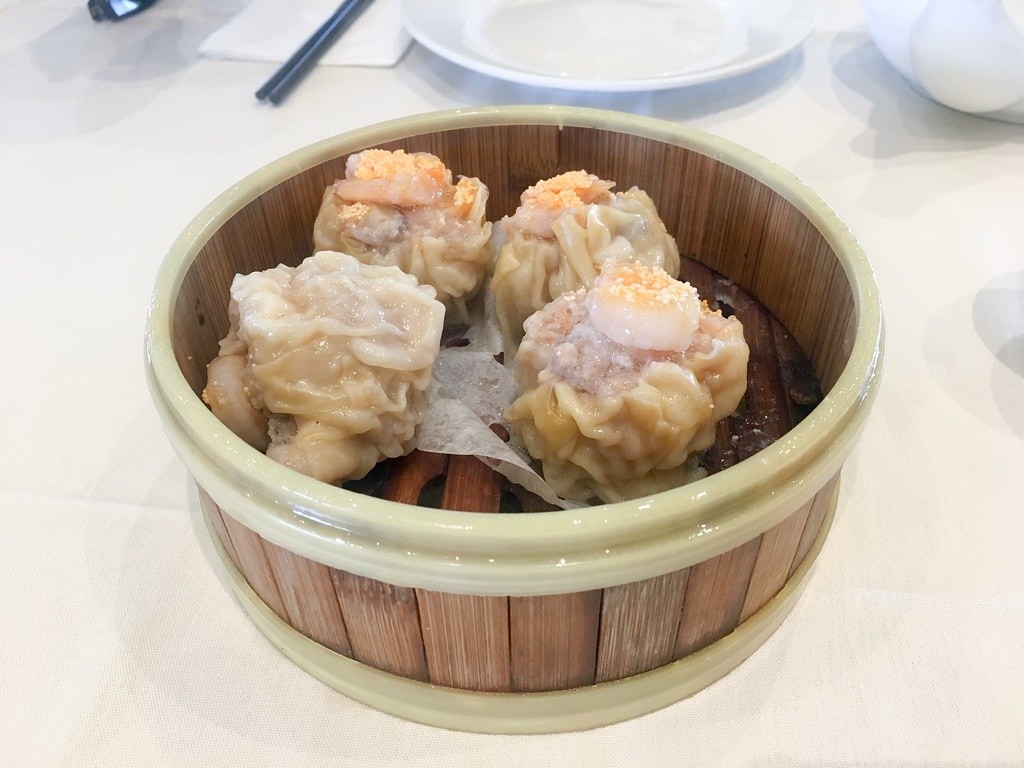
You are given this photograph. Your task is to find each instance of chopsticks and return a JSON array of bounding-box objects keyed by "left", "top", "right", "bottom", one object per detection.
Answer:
[{"left": 254, "top": 0, "right": 374, "bottom": 104}]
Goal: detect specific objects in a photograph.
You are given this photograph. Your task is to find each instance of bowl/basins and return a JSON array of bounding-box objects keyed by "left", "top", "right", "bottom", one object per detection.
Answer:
[{"left": 144, "top": 103, "right": 880, "bottom": 738}]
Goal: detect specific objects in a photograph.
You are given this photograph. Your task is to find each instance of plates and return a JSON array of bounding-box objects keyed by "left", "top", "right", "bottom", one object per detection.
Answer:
[{"left": 392, "top": 0, "right": 819, "bottom": 91}]
